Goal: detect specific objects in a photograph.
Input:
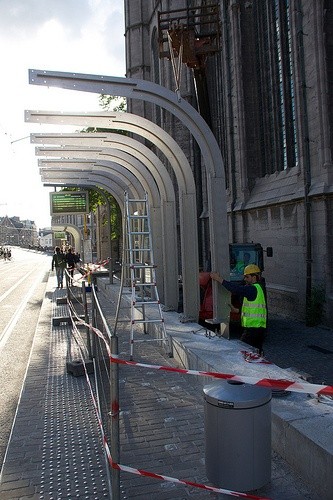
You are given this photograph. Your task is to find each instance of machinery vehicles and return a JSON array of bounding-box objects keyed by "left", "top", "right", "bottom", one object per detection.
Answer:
[{"left": 197, "top": 239, "right": 274, "bottom": 336}]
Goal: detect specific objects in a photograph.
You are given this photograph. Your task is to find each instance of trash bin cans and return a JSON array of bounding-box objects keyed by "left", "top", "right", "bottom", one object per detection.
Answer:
[{"left": 200, "top": 378, "right": 273, "bottom": 493}]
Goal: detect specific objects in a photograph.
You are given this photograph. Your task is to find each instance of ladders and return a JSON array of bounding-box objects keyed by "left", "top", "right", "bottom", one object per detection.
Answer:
[{"left": 112, "top": 189, "right": 172, "bottom": 360}]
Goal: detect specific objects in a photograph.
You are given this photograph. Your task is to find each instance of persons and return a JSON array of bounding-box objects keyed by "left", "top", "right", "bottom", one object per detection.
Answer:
[
  {"left": 210, "top": 265, "right": 267, "bottom": 354},
  {"left": 64, "top": 249, "right": 76, "bottom": 286},
  {"left": 233, "top": 251, "right": 254, "bottom": 272},
  {"left": 51, "top": 246, "right": 65, "bottom": 288}
]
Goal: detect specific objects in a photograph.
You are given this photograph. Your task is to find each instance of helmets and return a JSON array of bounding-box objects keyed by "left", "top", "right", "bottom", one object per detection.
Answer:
[{"left": 243, "top": 264, "right": 261, "bottom": 276}]
